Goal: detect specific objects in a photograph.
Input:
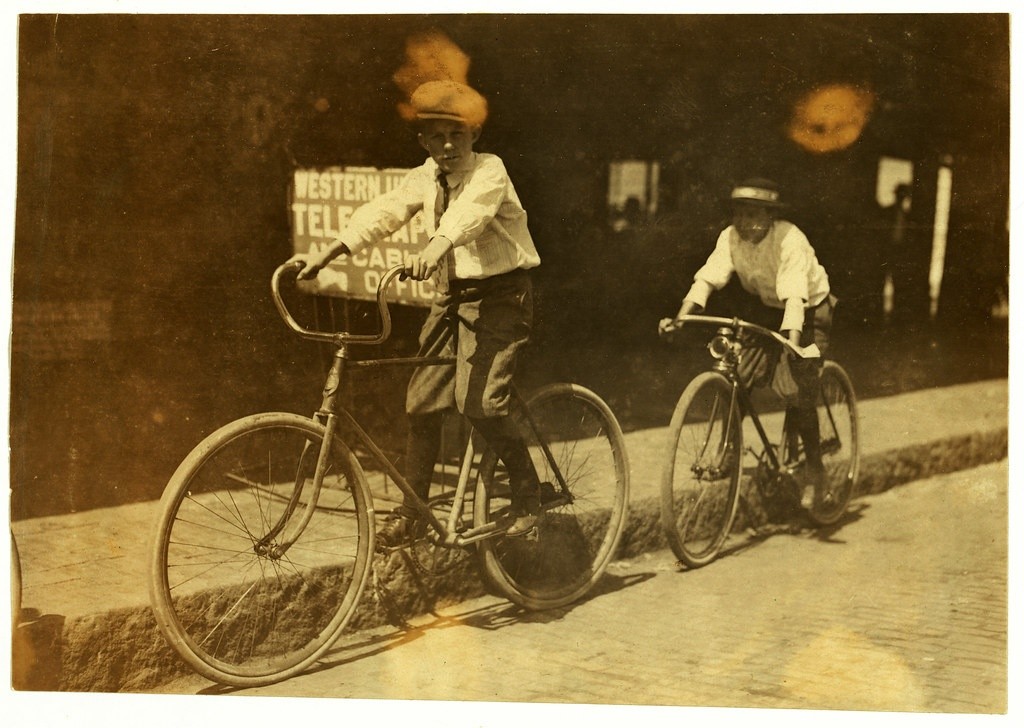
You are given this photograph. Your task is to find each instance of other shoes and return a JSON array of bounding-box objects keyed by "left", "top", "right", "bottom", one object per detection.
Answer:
[
  {"left": 505, "top": 488, "right": 547, "bottom": 537},
  {"left": 802, "top": 468, "right": 828, "bottom": 508},
  {"left": 702, "top": 451, "right": 734, "bottom": 480},
  {"left": 375, "top": 514, "right": 427, "bottom": 551}
]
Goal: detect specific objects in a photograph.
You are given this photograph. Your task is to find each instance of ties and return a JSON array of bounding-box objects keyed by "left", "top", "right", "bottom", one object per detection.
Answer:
[{"left": 435, "top": 175, "right": 450, "bottom": 307}]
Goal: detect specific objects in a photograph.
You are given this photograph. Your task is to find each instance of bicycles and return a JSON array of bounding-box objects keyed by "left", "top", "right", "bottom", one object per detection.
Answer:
[
  {"left": 654, "top": 312, "right": 861, "bottom": 567},
  {"left": 143, "top": 262, "right": 630, "bottom": 686}
]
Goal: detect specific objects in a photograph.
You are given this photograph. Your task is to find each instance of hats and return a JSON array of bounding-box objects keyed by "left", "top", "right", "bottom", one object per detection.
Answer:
[
  {"left": 411, "top": 81, "right": 487, "bottom": 125},
  {"left": 729, "top": 175, "right": 784, "bottom": 208}
]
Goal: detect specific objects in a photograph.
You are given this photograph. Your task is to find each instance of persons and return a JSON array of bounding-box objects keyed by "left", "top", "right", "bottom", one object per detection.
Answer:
[
  {"left": 283, "top": 80, "right": 546, "bottom": 551},
  {"left": 658, "top": 176, "right": 834, "bottom": 506}
]
